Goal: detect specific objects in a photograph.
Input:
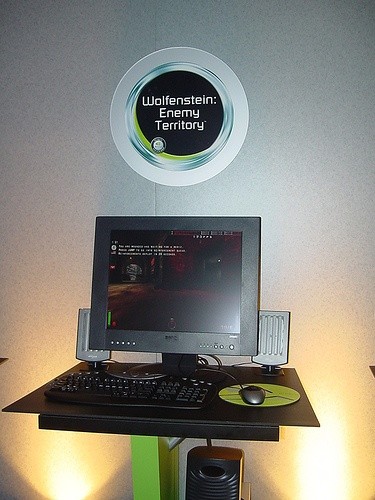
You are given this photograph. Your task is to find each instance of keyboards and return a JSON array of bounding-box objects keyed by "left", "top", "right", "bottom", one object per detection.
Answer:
[{"left": 44, "top": 372, "right": 217, "bottom": 409}]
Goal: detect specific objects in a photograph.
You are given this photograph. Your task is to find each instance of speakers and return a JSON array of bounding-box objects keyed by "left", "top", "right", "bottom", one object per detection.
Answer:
[
  {"left": 251, "top": 310, "right": 290, "bottom": 376},
  {"left": 76, "top": 308, "right": 111, "bottom": 371}
]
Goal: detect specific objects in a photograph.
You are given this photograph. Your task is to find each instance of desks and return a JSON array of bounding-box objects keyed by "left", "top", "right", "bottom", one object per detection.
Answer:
[{"left": 1, "top": 362, "right": 320, "bottom": 500}]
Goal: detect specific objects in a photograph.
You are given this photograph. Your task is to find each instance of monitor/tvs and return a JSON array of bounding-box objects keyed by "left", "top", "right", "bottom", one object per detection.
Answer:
[{"left": 89, "top": 216, "right": 261, "bottom": 382}]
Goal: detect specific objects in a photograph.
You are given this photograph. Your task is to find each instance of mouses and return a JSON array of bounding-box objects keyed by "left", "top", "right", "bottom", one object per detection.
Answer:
[{"left": 240, "top": 386, "right": 265, "bottom": 405}]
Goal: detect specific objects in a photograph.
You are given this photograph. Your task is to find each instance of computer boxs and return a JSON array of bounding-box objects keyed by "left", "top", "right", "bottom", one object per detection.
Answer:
[{"left": 186, "top": 446, "right": 245, "bottom": 500}]
